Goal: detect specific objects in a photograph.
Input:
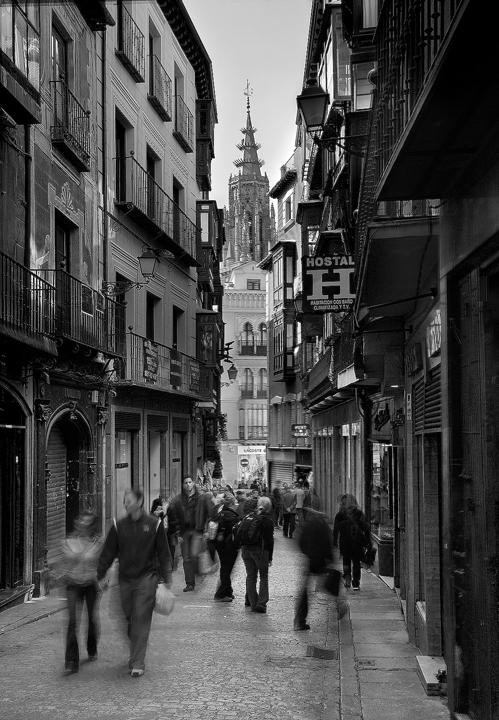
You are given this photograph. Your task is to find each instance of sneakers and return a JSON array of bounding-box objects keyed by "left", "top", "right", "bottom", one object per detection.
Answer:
[
  {"left": 130, "top": 663, "right": 145, "bottom": 678},
  {"left": 353, "top": 584, "right": 360, "bottom": 592},
  {"left": 87, "top": 646, "right": 98, "bottom": 661},
  {"left": 294, "top": 623, "right": 310, "bottom": 631},
  {"left": 182, "top": 582, "right": 195, "bottom": 592},
  {"left": 63, "top": 666, "right": 79, "bottom": 674},
  {"left": 245, "top": 593, "right": 266, "bottom": 614},
  {"left": 344, "top": 574, "right": 351, "bottom": 589},
  {"left": 213, "top": 594, "right": 233, "bottom": 602}
]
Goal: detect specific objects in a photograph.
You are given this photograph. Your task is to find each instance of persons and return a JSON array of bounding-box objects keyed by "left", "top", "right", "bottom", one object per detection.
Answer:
[
  {"left": 61, "top": 509, "right": 111, "bottom": 676},
  {"left": 97, "top": 490, "right": 173, "bottom": 677},
  {"left": 149, "top": 476, "right": 373, "bottom": 631}
]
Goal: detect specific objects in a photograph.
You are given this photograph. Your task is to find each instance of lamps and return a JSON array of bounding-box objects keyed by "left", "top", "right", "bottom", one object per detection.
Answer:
[
  {"left": 224, "top": 357, "right": 238, "bottom": 382},
  {"left": 295, "top": 79, "right": 366, "bottom": 157},
  {"left": 294, "top": 290, "right": 327, "bottom": 323},
  {"left": 102, "top": 247, "right": 160, "bottom": 297}
]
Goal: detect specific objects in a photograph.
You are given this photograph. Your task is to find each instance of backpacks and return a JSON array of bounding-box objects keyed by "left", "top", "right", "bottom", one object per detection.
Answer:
[
  {"left": 202, "top": 511, "right": 225, "bottom": 542},
  {"left": 237, "top": 511, "right": 267, "bottom": 546}
]
